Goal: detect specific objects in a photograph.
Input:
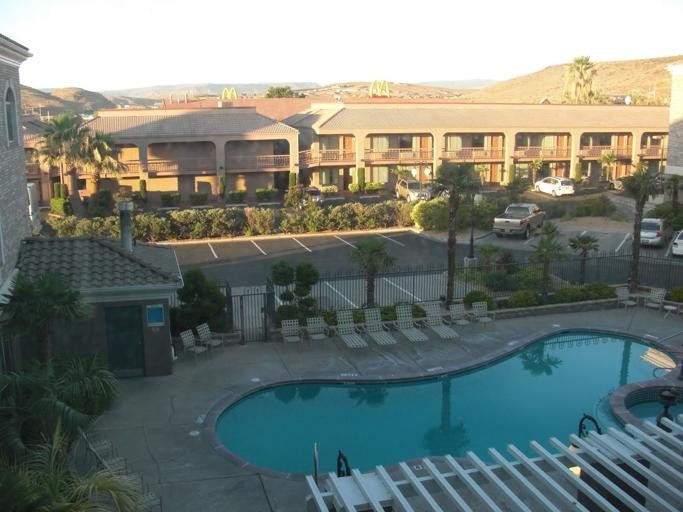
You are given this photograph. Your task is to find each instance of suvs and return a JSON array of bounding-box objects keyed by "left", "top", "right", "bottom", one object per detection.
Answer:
[
  {"left": 535, "top": 176, "right": 577, "bottom": 198},
  {"left": 395, "top": 178, "right": 432, "bottom": 204}
]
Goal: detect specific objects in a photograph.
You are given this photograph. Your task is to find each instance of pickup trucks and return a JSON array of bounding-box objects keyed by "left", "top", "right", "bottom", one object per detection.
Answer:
[{"left": 491, "top": 202, "right": 546, "bottom": 241}]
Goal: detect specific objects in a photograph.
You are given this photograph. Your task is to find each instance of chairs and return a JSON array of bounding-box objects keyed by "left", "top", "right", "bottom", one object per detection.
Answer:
[
  {"left": 280, "top": 298, "right": 496, "bottom": 355},
  {"left": 73, "top": 422, "right": 165, "bottom": 511},
  {"left": 614, "top": 284, "right": 668, "bottom": 319},
  {"left": 177, "top": 321, "right": 222, "bottom": 367}
]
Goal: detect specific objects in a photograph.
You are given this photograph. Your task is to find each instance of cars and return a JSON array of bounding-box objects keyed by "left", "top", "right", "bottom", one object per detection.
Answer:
[
  {"left": 608, "top": 174, "right": 669, "bottom": 193},
  {"left": 631, "top": 218, "right": 683, "bottom": 258}
]
